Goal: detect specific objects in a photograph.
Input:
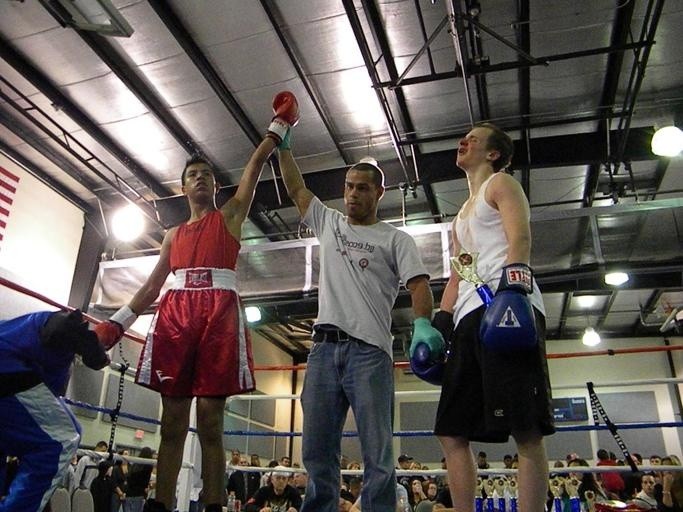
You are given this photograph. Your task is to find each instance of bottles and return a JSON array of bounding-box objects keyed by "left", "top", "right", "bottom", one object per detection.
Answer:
[
  {"left": 225, "top": 489, "right": 235, "bottom": 511},
  {"left": 474, "top": 496, "right": 580, "bottom": 512}
]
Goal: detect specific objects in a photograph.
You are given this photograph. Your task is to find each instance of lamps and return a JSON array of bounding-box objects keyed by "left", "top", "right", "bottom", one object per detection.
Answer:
[
  {"left": 581, "top": 306, "right": 602, "bottom": 349},
  {"left": 637, "top": 60, "right": 683, "bottom": 156}
]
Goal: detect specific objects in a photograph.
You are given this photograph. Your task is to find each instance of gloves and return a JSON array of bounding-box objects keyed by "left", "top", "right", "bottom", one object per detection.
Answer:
[
  {"left": 410, "top": 311, "right": 454, "bottom": 384},
  {"left": 478, "top": 262, "right": 538, "bottom": 349},
  {"left": 94, "top": 304, "right": 136, "bottom": 349},
  {"left": 266, "top": 90, "right": 299, "bottom": 144}
]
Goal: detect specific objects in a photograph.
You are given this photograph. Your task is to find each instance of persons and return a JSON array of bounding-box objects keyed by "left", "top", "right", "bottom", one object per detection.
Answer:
[
  {"left": 476, "top": 451, "right": 519, "bottom": 480},
  {"left": 0, "top": 306, "right": 110, "bottom": 511},
  {"left": 191, "top": 448, "right": 308, "bottom": 510},
  {"left": 410, "top": 124, "right": 556, "bottom": 511},
  {"left": 554, "top": 448, "right": 682, "bottom": 510},
  {"left": 95, "top": 89, "right": 300, "bottom": 512},
  {"left": 1, "top": 441, "right": 158, "bottom": 510},
  {"left": 472, "top": 475, "right": 583, "bottom": 511},
  {"left": 277, "top": 124, "right": 448, "bottom": 511},
  {"left": 338, "top": 455, "right": 364, "bottom": 511},
  {"left": 394, "top": 454, "right": 453, "bottom": 510}
]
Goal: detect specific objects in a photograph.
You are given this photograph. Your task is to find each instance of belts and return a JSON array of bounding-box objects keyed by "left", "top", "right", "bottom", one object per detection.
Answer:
[{"left": 311, "top": 326, "right": 351, "bottom": 344}]
